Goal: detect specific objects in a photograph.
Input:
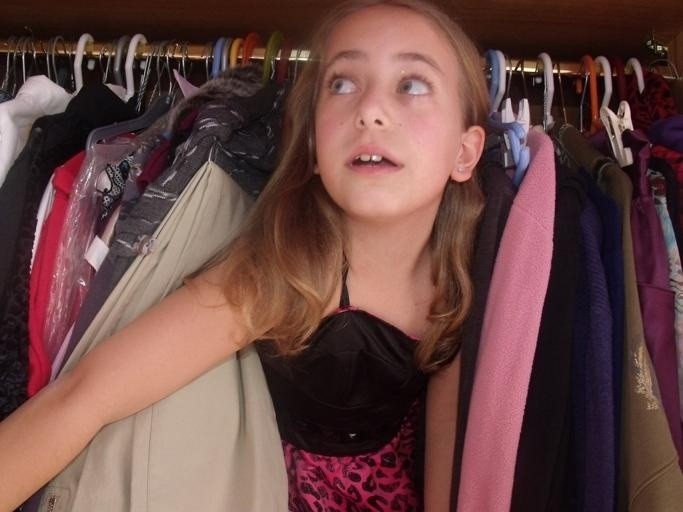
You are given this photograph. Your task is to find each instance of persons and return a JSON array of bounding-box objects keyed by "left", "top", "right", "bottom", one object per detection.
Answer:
[{"left": 1, "top": 1, "right": 493, "bottom": 512}]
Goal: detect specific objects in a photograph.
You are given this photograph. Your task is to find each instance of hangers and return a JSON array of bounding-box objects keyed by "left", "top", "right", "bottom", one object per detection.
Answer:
[{"left": 481, "top": 47, "right": 683, "bottom": 183}]
